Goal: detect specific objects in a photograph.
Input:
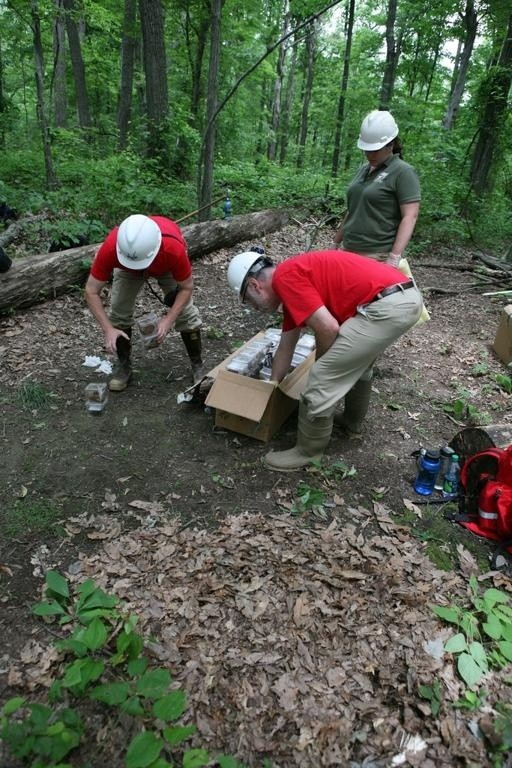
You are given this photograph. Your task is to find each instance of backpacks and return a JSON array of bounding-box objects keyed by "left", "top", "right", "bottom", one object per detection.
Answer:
[{"left": 458, "top": 444, "right": 512, "bottom": 554}]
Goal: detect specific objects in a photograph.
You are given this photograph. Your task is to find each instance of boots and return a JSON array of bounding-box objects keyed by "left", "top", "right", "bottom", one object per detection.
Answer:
[
  {"left": 264, "top": 396, "right": 335, "bottom": 472},
  {"left": 180, "top": 328, "right": 209, "bottom": 385},
  {"left": 334, "top": 376, "right": 373, "bottom": 434},
  {"left": 108, "top": 325, "right": 133, "bottom": 391}
]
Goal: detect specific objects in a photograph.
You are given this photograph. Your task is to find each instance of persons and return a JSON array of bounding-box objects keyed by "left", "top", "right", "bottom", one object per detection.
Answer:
[
  {"left": 226, "top": 249, "right": 422, "bottom": 476},
  {"left": 332, "top": 109, "right": 420, "bottom": 271},
  {"left": 84, "top": 212, "right": 215, "bottom": 393}
]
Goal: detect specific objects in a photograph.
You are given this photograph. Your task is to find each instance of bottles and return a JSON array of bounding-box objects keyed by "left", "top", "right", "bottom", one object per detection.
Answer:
[
  {"left": 414, "top": 447, "right": 460, "bottom": 498},
  {"left": 225, "top": 197, "right": 231, "bottom": 220}
]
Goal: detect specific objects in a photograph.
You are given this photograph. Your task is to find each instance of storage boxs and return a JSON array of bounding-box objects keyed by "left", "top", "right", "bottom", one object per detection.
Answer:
[
  {"left": 492, "top": 304, "right": 512, "bottom": 369},
  {"left": 183, "top": 327, "right": 317, "bottom": 443}
]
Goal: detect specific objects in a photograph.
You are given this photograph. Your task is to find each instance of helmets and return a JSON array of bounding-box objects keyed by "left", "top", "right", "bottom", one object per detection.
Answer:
[
  {"left": 357, "top": 109, "right": 400, "bottom": 152},
  {"left": 115, "top": 213, "right": 162, "bottom": 270},
  {"left": 227, "top": 251, "right": 267, "bottom": 302}
]
